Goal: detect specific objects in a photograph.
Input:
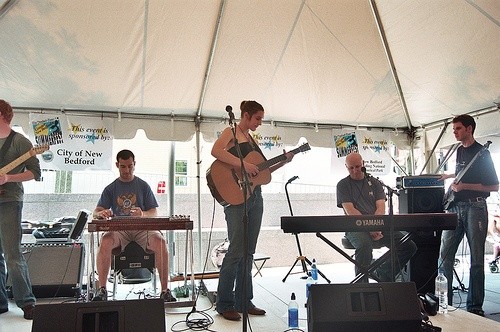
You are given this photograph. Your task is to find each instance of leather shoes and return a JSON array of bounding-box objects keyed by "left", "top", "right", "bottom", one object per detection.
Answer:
[
  {"left": 222, "top": 311, "right": 241, "bottom": 320},
  {"left": 248, "top": 307, "right": 266, "bottom": 315}
]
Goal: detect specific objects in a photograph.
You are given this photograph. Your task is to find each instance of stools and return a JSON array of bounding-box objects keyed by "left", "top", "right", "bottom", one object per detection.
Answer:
[
  {"left": 342, "top": 239, "right": 382, "bottom": 258},
  {"left": 252, "top": 256, "right": 271, "bottom": 278}
]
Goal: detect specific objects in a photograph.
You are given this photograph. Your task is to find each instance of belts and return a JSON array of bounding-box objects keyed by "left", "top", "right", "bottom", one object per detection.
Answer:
[{"left": 463, "top": 198, "right": 481, "bottom": 202}]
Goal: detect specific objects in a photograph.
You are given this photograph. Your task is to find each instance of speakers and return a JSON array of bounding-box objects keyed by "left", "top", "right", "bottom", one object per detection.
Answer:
[
  {"left": 399, "top": 187, "right": 446, "bottom": 295},
  {"left": 305, "top": 281, "right": 422, "bottom": 332},
  {"left": 5, "top": 244, "right": 85, "bottom": 298},
  {"left": 32, "top": 298, "right": 167, "bottom": 332}
]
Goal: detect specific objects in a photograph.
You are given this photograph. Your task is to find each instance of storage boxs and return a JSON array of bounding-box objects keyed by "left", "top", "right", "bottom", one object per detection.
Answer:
[{"left": 35, "top": 211, "right": 90, "bottom": 243}]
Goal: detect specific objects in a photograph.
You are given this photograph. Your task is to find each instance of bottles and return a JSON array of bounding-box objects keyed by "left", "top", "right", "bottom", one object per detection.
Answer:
[
  {"left": 310, "top": 258, "right": 318, "bottom": 284},
  {"left": 288, "top": 292, "right": 299, "bottom": 329},
  {"left": 435, "top": 267, "right": 448, "bottom": 314},
  {"left": 305, "top": 272, "right": 315, "bottom": 301}
]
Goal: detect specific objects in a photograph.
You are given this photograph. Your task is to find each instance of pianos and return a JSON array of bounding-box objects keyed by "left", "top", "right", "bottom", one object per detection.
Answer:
[
  {"left": 281, "top": 213, "right": 458, "bottom": 284},
  {"left": 88, "top": 215, "right": 196, "bottom": 307}
]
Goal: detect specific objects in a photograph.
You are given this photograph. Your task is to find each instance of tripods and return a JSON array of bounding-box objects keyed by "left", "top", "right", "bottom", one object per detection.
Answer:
[{"left": 282, "top": 185, "right": 331, "bottom": 283}]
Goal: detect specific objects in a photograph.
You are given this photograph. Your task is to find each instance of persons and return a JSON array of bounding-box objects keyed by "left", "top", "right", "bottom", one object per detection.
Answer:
[
  {"left": 211, "top": 100, "right": 295, "bottom": 320},
  {"left": 0, "top": 99, "right": 41, "bottom": 318},
  {"left": 439, "top": 114, "right": 500, "bottom": 313},
  {"left": 337, "top": 152, "right": 417, "bottom": 281},
  {"left": 91, "top": 149, "right": 179, "bottom": 302},
  {"left": 492, "top": 220, "right": 500, "bottom": 260}
]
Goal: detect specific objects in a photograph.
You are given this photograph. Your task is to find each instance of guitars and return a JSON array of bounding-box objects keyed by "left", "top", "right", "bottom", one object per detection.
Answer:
[
  {"left": 443, "top": 141, "right": 492, "bottom": 210},
  {"left": 0, "top": 144, "right": 49, "bottom": 174},
  {"left": 206, "top": 142, "right": 311, "bottom": 208}
]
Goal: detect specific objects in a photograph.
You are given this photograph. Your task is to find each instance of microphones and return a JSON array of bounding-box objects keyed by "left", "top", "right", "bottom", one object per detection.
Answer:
[
  {"left": 226, "top": 106, "right": 235, "bottom": 119},
  {"left": 361, "top": 167, "right": 368, "bottom": 177}
]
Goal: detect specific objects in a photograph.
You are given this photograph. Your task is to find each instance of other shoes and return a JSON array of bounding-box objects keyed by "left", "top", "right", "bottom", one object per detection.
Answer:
[
  {"left": 22, "top": 305, "right": 34, "bottom": 320},
  {"left": 160, "top": 289, "right": 177, "bottom": 301},
  {"left": 93, "top": 287, "right": 107, "bottom": 301}
]
